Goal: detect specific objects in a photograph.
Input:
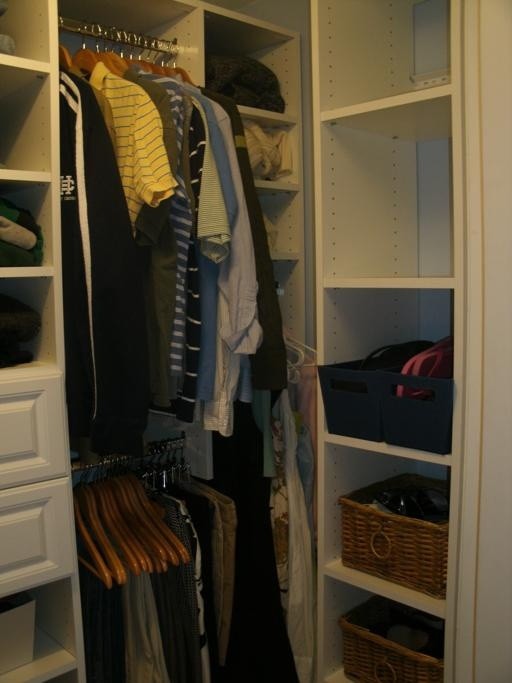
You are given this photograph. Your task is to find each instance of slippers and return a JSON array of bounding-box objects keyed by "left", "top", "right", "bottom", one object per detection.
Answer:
[
  {"left": 360, "top": 340, "right": 434, "bottom": 373},
  {"left": 397, "top": 337, "right": 453, "bottom": 399}
]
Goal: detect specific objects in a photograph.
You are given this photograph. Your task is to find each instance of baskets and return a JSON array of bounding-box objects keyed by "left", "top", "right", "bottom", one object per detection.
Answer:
[
  {"left": 338, "top": 595, "right": 443, "bottom": 682},
  {"left": 337, "top": 472, "right": 447, "bottom": 600}
]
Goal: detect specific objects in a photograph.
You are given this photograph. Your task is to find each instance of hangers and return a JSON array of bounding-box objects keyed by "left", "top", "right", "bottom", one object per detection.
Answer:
[
  {"left": 70, "top": 438, "right": 192, "bottom": 589},
  {"left": 282, "top": 325, "right": 324, "bottom": 385},
  {"left": 57, "top": 16, "right": 200, "bottom": 90}
]
